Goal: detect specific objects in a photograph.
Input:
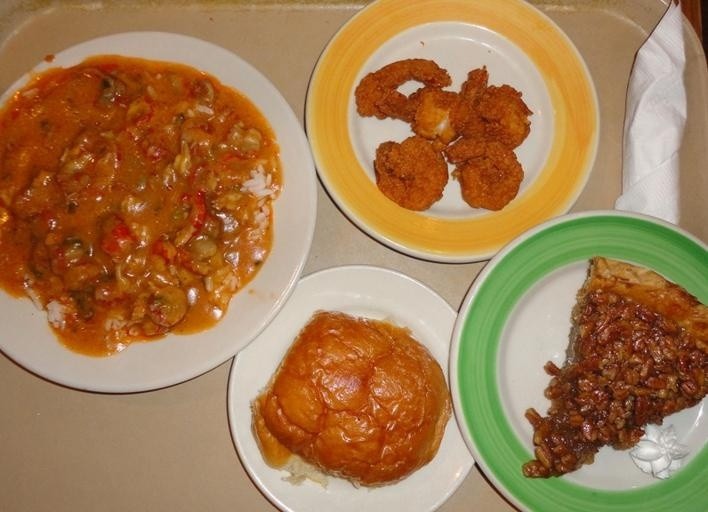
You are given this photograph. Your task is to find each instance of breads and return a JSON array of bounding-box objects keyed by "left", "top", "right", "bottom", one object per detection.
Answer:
[{"left": 251, "top": 313, "right": 452, "bottom": 488}]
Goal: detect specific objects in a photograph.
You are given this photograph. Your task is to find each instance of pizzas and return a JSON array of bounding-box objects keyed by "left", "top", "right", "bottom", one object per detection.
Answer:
[{"left": 523, "top": 255, "right": 708, "bottom": 478}]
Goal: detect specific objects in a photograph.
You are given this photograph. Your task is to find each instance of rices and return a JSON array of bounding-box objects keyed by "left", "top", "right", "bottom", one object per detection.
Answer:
[{"left": 47, "top": 103, "right": 275, "bottom": 338}]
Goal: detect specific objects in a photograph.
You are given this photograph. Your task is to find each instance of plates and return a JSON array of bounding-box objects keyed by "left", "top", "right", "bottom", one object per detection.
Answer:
[
  {"left": 448, "top": 209, "right": 706, "bottom": 510},
  {"left": 305, "top": 1, "right": 601, "bottom": 266},
  {"left": 222, "top": 263, "right": 476, "bottom": 511},
  {"left": 1, "top": 27, "right": 320, "bottom": 396}
]
